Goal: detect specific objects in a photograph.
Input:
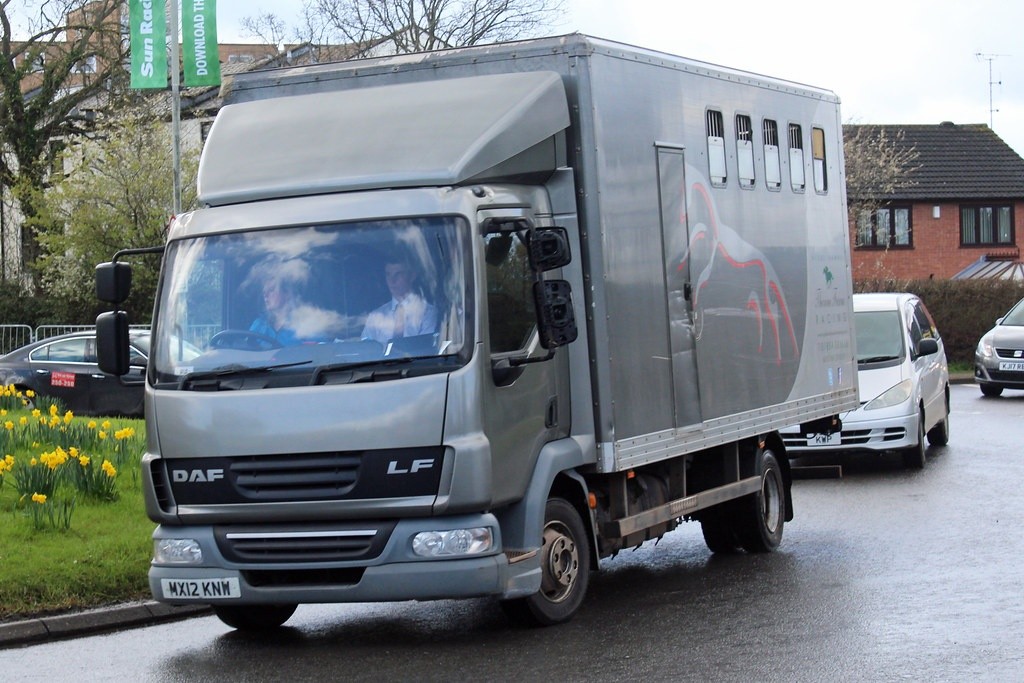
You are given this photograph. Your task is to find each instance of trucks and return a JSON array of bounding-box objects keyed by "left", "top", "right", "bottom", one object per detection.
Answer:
[{"left": 95, "top": 30, "right": 859, "bottom": 632}]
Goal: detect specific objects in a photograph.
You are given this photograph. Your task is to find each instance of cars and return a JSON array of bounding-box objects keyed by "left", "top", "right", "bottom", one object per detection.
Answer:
[
  {"left": 777, "top": 292, "right": 951, "bottom": 470},
  {"left": 0, "top": 328, "right": 205, "bottom": 420},
  {"left": 974, "top": 298, "right": 1024, "bottom": 398}
]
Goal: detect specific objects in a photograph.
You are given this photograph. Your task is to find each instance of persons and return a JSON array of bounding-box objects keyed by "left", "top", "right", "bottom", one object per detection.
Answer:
[
  {"left": 246, "top": 268, "right": 329, "bottom": 347},
  {"left": 360, "top": 253, "right": 438, "bottom": 350}
]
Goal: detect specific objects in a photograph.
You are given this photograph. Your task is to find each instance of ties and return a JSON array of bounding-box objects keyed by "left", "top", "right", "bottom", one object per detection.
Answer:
[{"left": 392, "top": 305, "right": 404, "bottom": 338}]
